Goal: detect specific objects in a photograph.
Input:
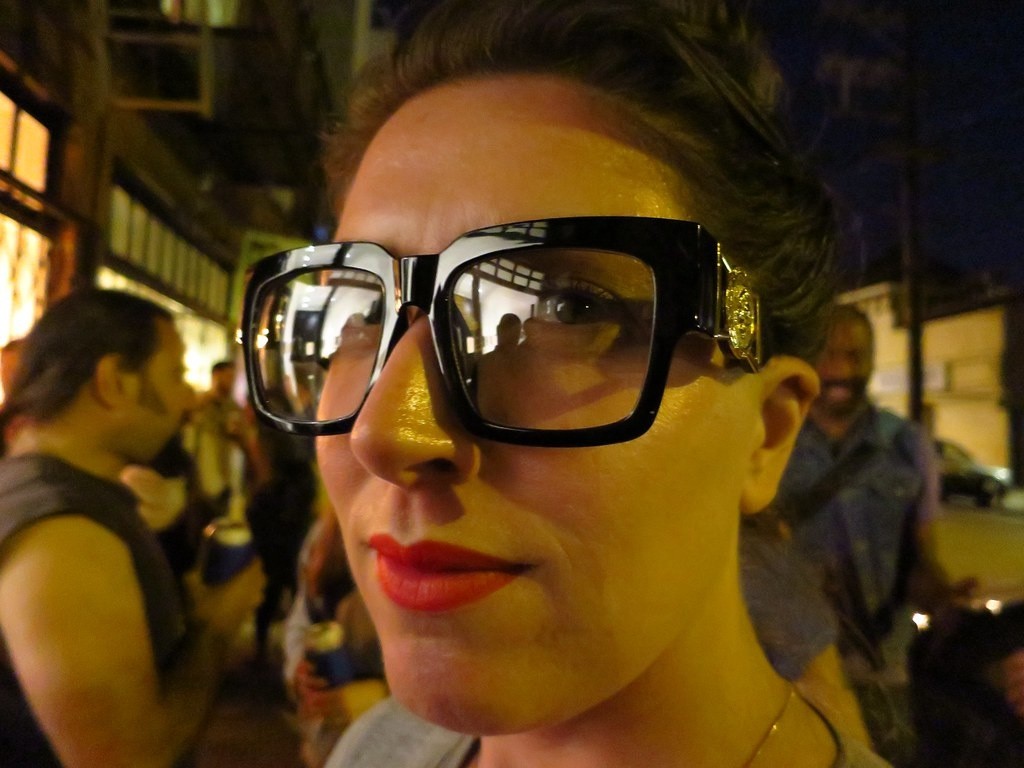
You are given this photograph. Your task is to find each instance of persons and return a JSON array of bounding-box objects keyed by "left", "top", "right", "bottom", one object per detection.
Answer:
[
  {"left": 0, "top": 288, "right": 391, "bottom": 768},
  {"left": 315, "top": 0, "right": 899, "bottom": 768},
  {"left": 736, "top": 304, "right": 1024, "bottom": 768}
]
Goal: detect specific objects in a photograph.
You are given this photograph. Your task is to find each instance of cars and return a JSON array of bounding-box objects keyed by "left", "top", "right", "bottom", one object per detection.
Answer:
[{"left": 934, "top": 441, "right": 1011, "bottom": 511}]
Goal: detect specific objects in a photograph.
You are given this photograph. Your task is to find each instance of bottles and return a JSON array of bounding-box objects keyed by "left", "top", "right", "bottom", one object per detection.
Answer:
[
  {"left": 201, "top": 518, "right": 251, "bottom": 587},
  {"left": 303, "top": 622, "right": 350, "bottom": 688}
]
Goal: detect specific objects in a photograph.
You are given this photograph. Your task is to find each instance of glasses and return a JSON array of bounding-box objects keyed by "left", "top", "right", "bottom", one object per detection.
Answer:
[{"left": 238, "top": 214, "right": 765, "bottom": 450}]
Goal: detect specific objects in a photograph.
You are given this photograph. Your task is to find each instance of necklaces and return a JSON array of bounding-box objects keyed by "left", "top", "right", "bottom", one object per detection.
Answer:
[{"left": 744, "top": 688, "right": 794, "bottom": 768}]
